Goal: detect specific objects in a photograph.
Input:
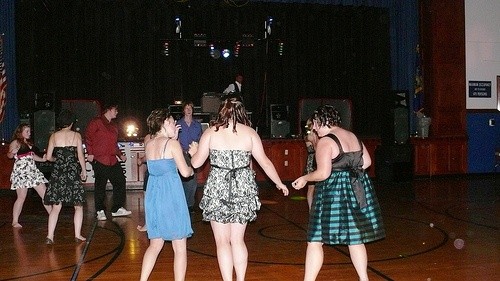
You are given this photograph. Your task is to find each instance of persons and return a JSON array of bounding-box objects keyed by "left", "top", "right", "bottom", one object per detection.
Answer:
[
  {"left": 84, "top": 99, "right": 132, "bottom": 220},
  {"left": 188, "top": 97, "right": 290, "bottom": 281},
  {"left": 45, "top": 110, "right": 87, "bottom": 246},
  {"left": 303, "top": 116, "right": 320, "bottom": 213},
  {"left": 6, "top": 121, "right": 54, "bottom": 228},
  {"left": 139, "top": 107, "right": 195, "bottom": 281},
  {"left": 221, "top": 75, "right": 244, "bottom": 98},
  {"left": 175, "top": 102, "right": 204, "bottom": 212},
  {"left": 292, "top": 104, "right": 386, "bottom": 281}
]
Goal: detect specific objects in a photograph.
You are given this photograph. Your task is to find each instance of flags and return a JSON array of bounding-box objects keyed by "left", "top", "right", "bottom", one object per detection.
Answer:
[
  {"left": 1, "top": 34, "right": 9, "bottom": 124},
  {"left": 412, "top": 41, "right": 426, "bottom": 116}
]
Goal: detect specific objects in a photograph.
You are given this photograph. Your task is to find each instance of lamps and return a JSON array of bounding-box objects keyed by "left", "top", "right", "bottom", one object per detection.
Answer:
[{"left": 223, "top": 48, "right": 232, "bottom": 59}]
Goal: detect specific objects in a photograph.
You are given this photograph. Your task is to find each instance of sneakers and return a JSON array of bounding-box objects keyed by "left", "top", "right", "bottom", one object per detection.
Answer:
[
  {"left": 96, "top": 210, "right": 108, "bottom": 221},
  {"left": 112, "top": 206, "right": 131, "bottom": 216}
]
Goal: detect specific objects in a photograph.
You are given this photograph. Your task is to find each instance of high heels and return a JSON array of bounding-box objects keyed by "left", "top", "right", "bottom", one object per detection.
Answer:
[
  {"left": 74, "top": 237, "right": 87, "bottom": 243},
  {"left": 45, "top": 238, "right": 55, "bottom": 245}
]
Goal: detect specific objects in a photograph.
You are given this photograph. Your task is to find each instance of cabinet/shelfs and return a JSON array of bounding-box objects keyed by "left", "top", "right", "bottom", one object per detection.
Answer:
[
  {"left": 253, "top": 138, "right": 379, "bottom": 180},
  {"left": 409, "top": 137, "right": 467, "bottom": 176}
]
